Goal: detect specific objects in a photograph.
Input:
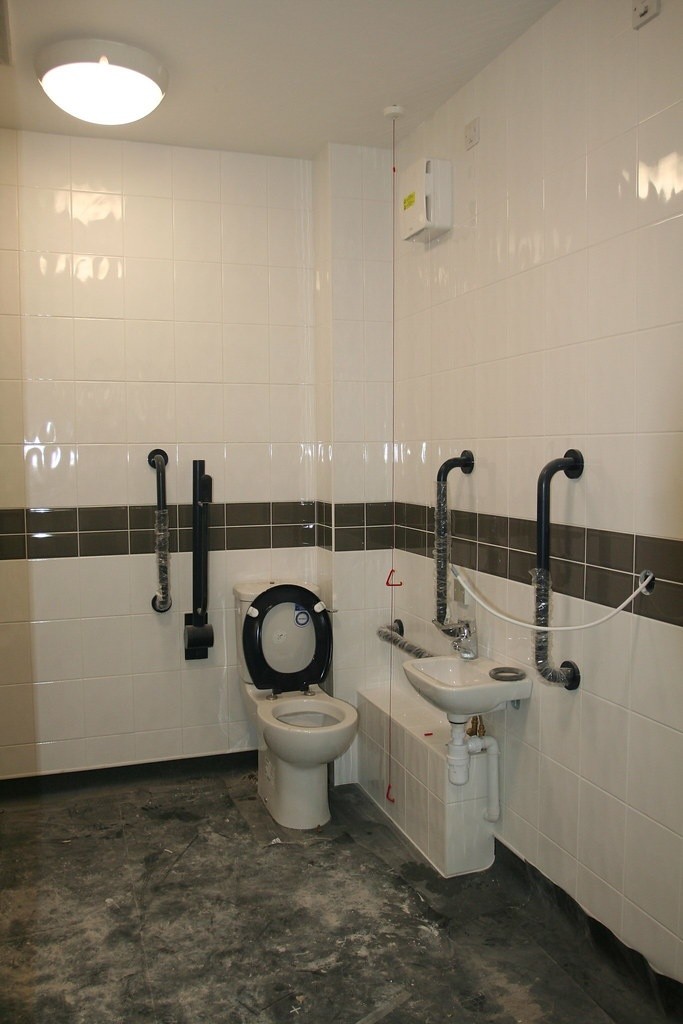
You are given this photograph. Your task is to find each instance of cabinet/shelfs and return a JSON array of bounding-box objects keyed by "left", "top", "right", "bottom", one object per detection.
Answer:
[{"left": 356, "top": 685, "right": 495, "bottom": 879}]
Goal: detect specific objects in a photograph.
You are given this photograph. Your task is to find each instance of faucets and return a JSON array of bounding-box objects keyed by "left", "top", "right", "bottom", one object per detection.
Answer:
[{"left": 452, "top": 636, "right": 477, "bottom": 660}]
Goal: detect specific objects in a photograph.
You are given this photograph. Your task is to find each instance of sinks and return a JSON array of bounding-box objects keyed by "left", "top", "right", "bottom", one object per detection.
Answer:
[{"left": 402, "top": 646, "right": 535, "bottom": 714}]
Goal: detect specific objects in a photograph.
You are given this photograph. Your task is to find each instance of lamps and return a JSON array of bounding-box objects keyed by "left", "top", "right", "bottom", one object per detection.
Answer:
[{"left": 36, "top": 36, "right": 169, "bottom": 129}]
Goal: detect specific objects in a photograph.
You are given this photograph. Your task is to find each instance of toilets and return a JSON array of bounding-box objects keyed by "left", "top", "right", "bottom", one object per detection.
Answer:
[{"left": 232, "top": 578, "right": 360, "bottom": 829}]
